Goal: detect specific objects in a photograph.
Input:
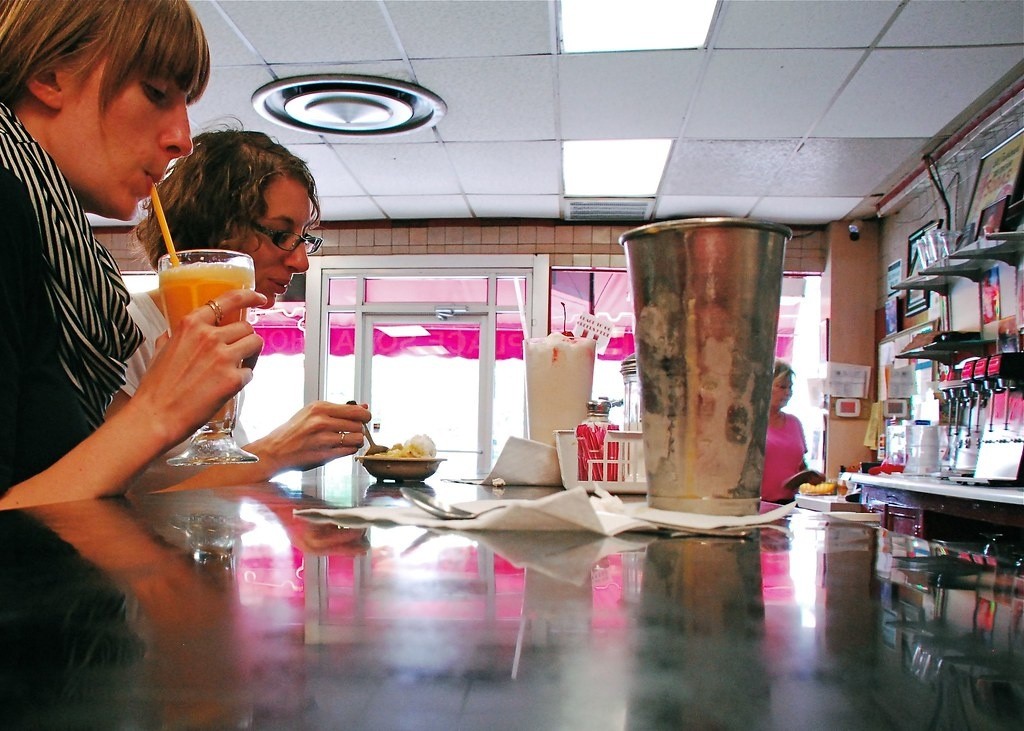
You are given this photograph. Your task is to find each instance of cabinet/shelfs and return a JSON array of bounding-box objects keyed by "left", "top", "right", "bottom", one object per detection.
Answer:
[
  {"left": 891, "top": 232, "right": 1024, "bottom": 366},
  {"left": 840, "top": 473, "right": 1024, "bottom": 540}
]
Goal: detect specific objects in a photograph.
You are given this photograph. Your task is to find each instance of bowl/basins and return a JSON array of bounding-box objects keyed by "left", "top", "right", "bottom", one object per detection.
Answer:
[{"left": 355, "top": 456, "right": 448, "bottom": 483}]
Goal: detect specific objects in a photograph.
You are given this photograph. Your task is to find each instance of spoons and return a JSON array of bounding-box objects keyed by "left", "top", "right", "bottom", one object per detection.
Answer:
[{"left": 346, "top": 400, "right": 388, "bottom": 456}]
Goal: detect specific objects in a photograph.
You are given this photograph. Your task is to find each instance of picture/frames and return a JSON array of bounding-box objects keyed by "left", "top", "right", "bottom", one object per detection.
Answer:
[
  {"left": 955, "top": 128, "right": 1023, "bottom": 248},
  {"left": 904, "top": 218, "right": 943, "bottom": 318},
  {"left": 884, "top": 297, "right": 898, "bottom": 337}
]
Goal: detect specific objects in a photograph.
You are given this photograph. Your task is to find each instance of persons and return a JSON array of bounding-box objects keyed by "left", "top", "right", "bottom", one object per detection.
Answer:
[
  {"left": 108, "top": 128, "right": 372, "bottom": 495},
  {"left": 0, "top": 480, "right": 369, "bottom": 731},
  {"left": 760, "top": 361, "right": 808, "bottom": 506},
  {"left": 0, "top": 0, "right": 269, "bottom": 509}
]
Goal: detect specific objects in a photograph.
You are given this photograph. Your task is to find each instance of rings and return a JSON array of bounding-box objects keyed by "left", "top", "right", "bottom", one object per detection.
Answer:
[
  {"left": 339, "top": 431, "right": 345, "bottom": 447},
  {"left": 206, "top": 299, "right": 224, "bottom": 323}
]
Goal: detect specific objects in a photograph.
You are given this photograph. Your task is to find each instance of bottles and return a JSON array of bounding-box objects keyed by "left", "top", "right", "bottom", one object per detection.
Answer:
[
  {"left": 619, "top": 352, "right": 647, "bottom": 485},
  {"left": 578, "top": 399, "right": 618, "bottom": 482}
]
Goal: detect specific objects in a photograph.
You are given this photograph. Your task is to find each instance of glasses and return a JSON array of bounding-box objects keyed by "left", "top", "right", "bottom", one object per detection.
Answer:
[{"left": 235, "top": 217, "right": 324, "bottom": 254}]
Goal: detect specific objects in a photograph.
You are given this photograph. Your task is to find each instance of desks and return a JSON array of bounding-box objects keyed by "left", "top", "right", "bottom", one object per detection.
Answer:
[{"left": 0, "top": 478, "right": 1024, "bottom": 731}]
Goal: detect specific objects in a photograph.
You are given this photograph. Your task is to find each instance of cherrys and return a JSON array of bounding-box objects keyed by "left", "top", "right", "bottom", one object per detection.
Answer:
[{"left": 559, "top": 302, "right": 573, "bottom": 337}]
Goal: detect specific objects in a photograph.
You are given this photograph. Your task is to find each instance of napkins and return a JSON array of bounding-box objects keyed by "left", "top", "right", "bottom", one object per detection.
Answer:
[{"left": 294, "top": 437, "right": 796, "bottom": 536}]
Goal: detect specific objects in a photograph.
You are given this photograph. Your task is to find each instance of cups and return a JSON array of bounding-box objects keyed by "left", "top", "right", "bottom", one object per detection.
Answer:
[
  {"left": 158, "top": 248, "right": 260, "bottom": 467},
  {"left": 617, "top": 218, "right": 794, "bottom": 517},
  {"left": 522, "top": 337, "right": 596, "bottom": 448}
]
